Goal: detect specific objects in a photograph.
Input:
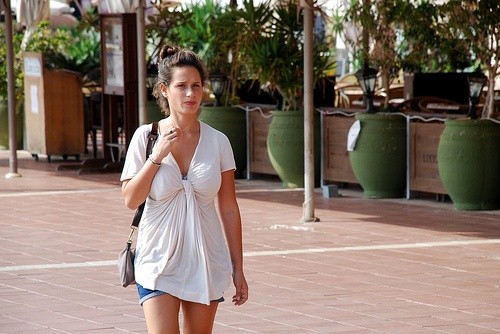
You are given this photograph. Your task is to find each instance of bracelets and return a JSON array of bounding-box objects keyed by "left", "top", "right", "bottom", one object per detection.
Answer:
[{"left": 148, "top": 154, "right": 161, "bottom": 167}]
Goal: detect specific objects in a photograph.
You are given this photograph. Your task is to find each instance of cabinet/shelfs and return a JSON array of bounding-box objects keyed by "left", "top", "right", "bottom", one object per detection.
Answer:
[{"left": 22, "top": 51, "right": 84, "bottom": 160}]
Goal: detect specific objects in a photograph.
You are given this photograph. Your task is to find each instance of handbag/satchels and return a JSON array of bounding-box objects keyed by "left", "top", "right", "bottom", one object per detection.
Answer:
[{"left": 119, "top": 243, "right": 137, "bottom": 286}]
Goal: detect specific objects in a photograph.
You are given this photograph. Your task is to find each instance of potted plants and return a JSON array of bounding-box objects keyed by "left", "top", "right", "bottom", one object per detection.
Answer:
[
  {"left": 442, "top": 0, "right": 500, "bottom": 211},
  {"left": 346, "top": 0, "right": 427, "bottom": 199},
  {"left": 175, "top": 3, "right": 250, "bottom": 179},
  {"left": 244, "top": 0, "right": 335, "bottom": 187}
]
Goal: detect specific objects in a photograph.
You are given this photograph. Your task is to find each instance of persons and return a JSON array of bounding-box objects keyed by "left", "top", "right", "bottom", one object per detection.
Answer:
[{"left": 119, "top": 42, "right": 250, "bottom": 334}]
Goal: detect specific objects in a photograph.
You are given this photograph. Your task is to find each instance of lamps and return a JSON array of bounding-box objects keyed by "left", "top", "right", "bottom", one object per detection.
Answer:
[{"left": 355, "top": 63, "right": 379, "bottom": 115}]
